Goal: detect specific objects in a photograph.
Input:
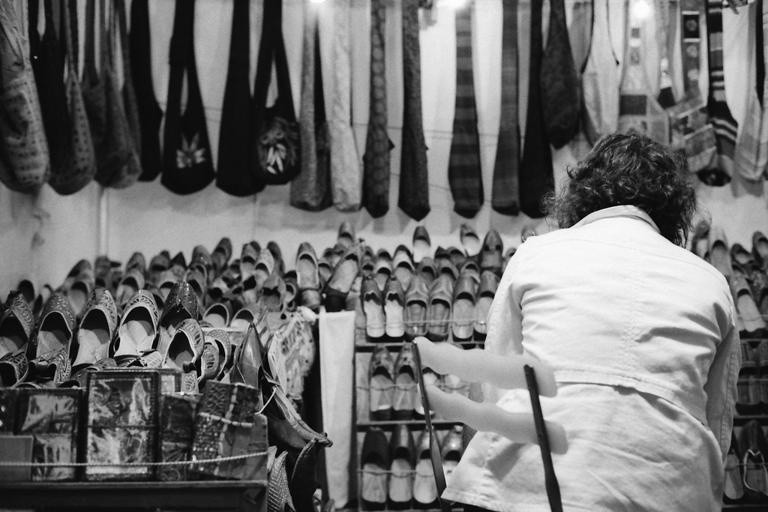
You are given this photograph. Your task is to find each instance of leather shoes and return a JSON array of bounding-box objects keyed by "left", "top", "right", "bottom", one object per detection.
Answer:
[
  {"left": 362, "top": 275, "right": 386, "bottom": 341},
  {"left": 388, "top": 424, "right": 413, "bottom": 510},
  {"left": 438, "top": 424, "right": 464, "bottom": 508},
  {"left": 452, "top": 269, "right": 475, "bottom": 341},
  {"left": 413, "top": 424, "right": 438, "bottom": 509},
  {"left": 0, "top": 221, "right": 360, "bottom": 512},
  {"left": 426, "top": 273, "right": 454, "bottom": 341},
  {"left": 383, "top": 275, "right": 405, "bottom": 341},
  {"left": 392, "top": 342, "right": 419, "bottom": 420},
  {"left": 474, "top": 270, "right": 497, "bottom": 341},
  {"left": 691, "top": 222, "right": 768, "bottom": 504},
  {"left": 369, "top": 345, "right": 395, "bottom": 421},
  {"left": 405, "top": 275, "right": 429, "bottom": 342},
  {"left": 362, "top": 222, "right": 516, "bottom": 288},
  {"left": 361, "top": 427, "right": 389, "bottom": 511}
]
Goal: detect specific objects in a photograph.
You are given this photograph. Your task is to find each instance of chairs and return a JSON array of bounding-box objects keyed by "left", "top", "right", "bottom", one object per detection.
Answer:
[{"left": 410, "top": 336, "right": 571, "bottom": 512}]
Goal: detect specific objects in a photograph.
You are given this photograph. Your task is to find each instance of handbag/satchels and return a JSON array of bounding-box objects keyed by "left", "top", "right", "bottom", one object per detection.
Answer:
[{"left": 0, "top": 0, "right": 767, "bottom": 222}]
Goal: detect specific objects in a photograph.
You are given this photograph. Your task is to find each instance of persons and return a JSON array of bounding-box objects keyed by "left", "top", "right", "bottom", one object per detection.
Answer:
[{"left": 441, "top": 129, "right": 744, "bottom": 512}]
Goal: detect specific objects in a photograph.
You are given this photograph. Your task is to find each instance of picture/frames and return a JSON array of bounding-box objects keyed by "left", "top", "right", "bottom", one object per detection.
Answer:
[{"left": 10, "top": 369, "right": 185, "bottom": 483}]
